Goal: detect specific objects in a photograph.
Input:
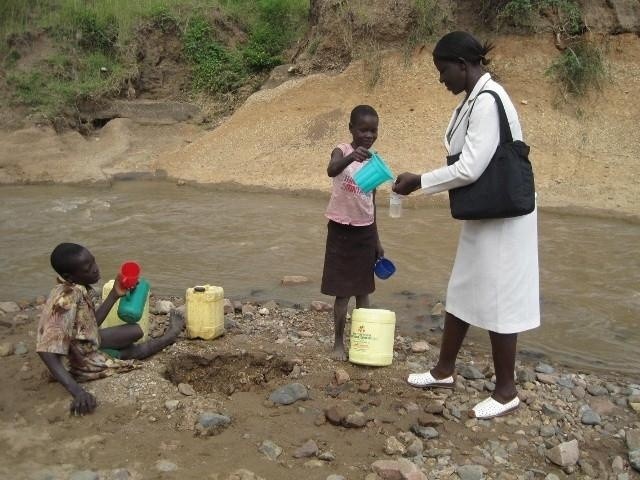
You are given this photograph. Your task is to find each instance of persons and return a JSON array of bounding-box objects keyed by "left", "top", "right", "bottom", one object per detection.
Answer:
[
  {"left": 31, "top": 240, "right": 188, "bottom": 420},
  {"left": 390, "top": 29, "right": 544, "bottom": 420},
  {"left": 316, "top": 102, "right": 386, "bottom": 364}
]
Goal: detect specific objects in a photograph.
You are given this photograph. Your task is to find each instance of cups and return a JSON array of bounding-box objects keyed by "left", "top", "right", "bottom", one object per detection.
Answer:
[
  {"left": 116, "top": 262, "right": 150, "bottom": 325},
  {"left": 374, "top": 255, "right": 395, "bottom": 280}
]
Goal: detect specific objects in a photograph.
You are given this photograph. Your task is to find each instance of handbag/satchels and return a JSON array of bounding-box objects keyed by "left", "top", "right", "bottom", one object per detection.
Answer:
[{"left": 446, "top": 89, "right": 535, "bottom": 220}]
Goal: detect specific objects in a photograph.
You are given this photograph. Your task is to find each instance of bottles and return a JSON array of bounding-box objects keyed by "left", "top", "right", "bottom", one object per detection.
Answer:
[{"left": 389, "top": 190, "right": 403, "bottom": 220}]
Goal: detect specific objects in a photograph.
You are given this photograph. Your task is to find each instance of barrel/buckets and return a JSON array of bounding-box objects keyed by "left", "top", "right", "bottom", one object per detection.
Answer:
[
  {"left": 118, "top": 278, "right": 151, "bottom": 323},
  {"left": 120, "top": 261, "right": 141, "bottom": 288},
  {"left": 185, "top": 285, "right": 225, "bottom": 340},
  {"left": 349, "top": 308, "right": 397, "bottom": 366},
  {"left": 102, "top": 280, "right": 149, "bottom": 344}
]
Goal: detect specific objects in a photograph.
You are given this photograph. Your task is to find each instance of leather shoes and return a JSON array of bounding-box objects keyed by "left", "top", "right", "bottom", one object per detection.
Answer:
[
  {"left": 468, "top": 393, "right": 521, "bottom": 421},
  {"left": 405, "top": 368, "right": 457, "bottom": 389}
]
